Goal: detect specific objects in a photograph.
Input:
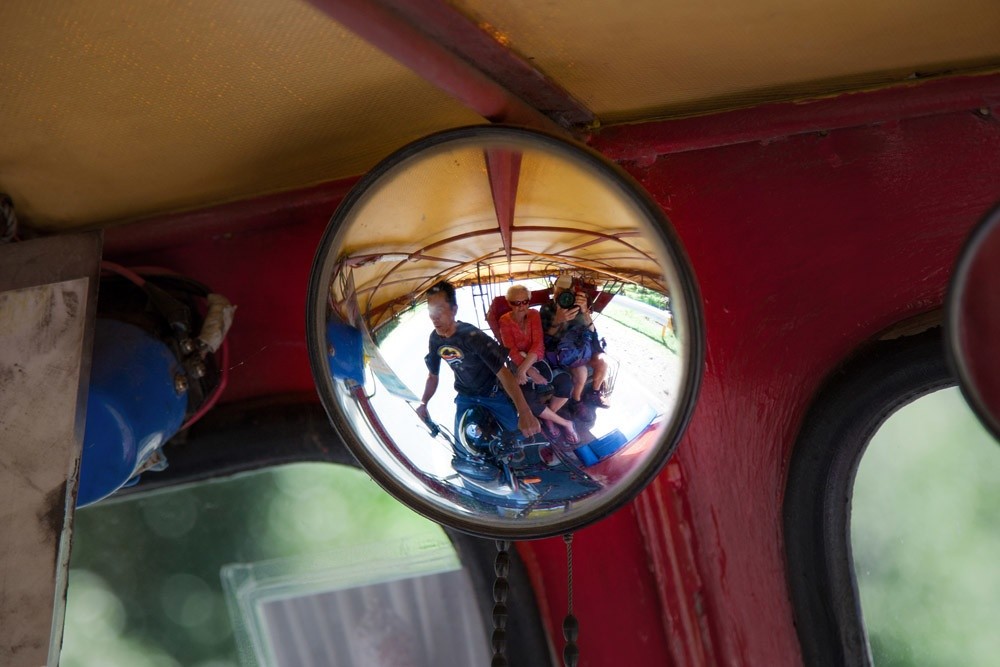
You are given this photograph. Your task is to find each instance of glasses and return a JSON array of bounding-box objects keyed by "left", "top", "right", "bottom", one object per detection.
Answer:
[{"left": 509, "top": 299, "right": 530, "bottom": 306}]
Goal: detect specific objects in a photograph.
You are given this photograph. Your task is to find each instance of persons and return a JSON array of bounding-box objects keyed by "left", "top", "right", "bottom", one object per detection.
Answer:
[
  {"left": 499, "top": 284, "right": 580, "bottom": 445},
  {"left": 540, "top": 274, "right": 608, "bottom": 422},
  {"left": 416, "top": 280, "right": 541, "bottom": 445}
]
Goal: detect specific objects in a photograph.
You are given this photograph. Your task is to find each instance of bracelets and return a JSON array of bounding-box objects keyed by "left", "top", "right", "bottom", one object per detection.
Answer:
[
  {"left": 586, "top": 321, "right": 593, "bottom": 328},
  {"left": 550, "top": 321, "right": 560, "bottom": 329}
]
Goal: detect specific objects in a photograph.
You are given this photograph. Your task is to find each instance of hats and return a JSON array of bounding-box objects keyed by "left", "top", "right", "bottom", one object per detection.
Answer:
[{"left": 555, "top": 274, "right": 575, "bottom": 289}]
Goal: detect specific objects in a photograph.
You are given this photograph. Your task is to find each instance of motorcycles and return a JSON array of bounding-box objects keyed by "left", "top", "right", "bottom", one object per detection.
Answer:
[{"left": 409, "top": 397, "right": 604, "bottom": 513}]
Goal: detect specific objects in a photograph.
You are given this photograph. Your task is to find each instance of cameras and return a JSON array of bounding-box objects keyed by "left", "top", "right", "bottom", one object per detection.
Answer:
[{"left": 555, "top": 291, "right": 576, "bottom": 309}]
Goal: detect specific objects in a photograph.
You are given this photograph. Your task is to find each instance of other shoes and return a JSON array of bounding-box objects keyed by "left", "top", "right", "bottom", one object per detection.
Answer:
[
  {"left": 572, "top": 399, "right": 593, "bottom": 422},
  {"left": 587, "top": 391, "right": 610, "bottom": 409}
]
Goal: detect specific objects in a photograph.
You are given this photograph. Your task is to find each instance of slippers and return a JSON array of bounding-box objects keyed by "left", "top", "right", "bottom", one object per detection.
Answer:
[
  {"left": 564, "top": 421, "right": 581, "bottom": 445},
  {"left": 542, "top": 421, "right": 561, "bottom": 439}
]
